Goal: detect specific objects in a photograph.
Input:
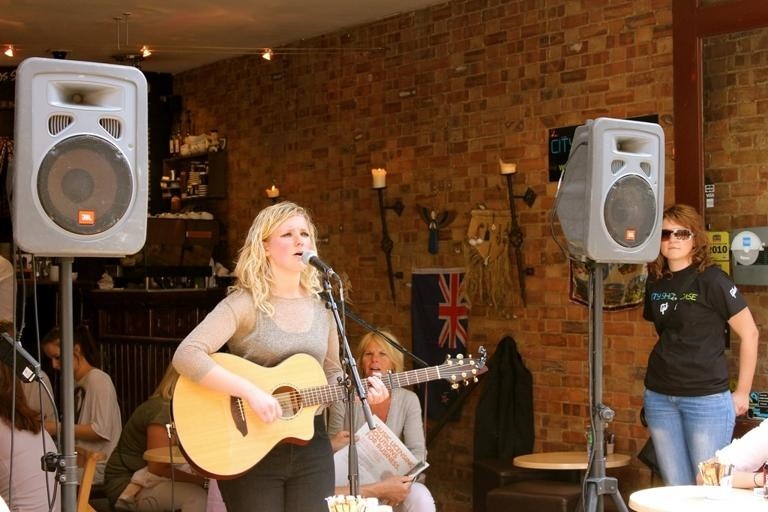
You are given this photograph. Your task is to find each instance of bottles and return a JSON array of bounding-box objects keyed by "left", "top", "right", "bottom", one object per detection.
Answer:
[{"left": 168, "top": 127, "right": 183, "bottom": 158}]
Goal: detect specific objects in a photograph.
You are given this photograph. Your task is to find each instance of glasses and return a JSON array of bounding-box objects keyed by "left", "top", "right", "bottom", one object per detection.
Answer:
[{"left": 662, "top": 229, "right": 695, "bottom": 241}]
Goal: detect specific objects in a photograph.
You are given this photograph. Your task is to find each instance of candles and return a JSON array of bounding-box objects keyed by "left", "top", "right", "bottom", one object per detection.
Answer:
[
  {"left": 371, "top": 168, "right": 387, "bottom": 188},
  {"left": 500, "top": 162, "right": 517, "bottom": 174},
  {"left": 266, "top": 188, "right": 279, "bottom": 198}
]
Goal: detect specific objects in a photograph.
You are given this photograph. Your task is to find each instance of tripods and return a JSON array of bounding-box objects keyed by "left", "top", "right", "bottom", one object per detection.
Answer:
[{"left": 575, "top": 263, "right": 628, "bottom": 511}]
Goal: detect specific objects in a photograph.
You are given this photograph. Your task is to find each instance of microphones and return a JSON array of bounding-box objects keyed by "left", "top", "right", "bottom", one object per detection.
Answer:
[{"left": 300, "top": 249, "right": 341, "bottom": 284}]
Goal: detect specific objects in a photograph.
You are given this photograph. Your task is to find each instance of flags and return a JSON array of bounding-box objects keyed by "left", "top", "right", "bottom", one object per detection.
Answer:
[{"left": 412, "top": 267, "right": 469, "bottom": 423}]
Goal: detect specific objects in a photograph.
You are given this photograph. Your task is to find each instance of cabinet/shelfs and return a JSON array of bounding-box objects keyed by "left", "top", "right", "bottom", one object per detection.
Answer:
[{"left": 90, "top": 149, "right": 227, "bottom": 426}]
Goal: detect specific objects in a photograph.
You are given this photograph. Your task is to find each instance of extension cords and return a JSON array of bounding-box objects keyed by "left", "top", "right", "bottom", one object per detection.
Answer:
[{"left": 0, "top": 331, "right": 42, "bottom": 383}]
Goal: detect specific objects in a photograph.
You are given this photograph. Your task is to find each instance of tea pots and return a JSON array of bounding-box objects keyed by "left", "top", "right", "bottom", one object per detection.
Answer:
[{"left": 205, "top": 128, "right": 226, "bottom": 151}]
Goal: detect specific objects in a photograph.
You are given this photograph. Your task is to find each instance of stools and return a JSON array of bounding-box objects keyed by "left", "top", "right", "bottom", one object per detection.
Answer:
[{"left": 472, "top": 460, "right": 583, "bottom": 511}]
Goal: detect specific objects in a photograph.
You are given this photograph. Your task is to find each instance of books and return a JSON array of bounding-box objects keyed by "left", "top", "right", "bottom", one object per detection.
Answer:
[{"left": 334, "top": 413, "right": 431, "bottom": 489}]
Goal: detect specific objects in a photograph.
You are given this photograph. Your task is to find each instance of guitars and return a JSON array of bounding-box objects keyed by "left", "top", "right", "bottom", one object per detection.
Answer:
[{"left": 170, "top": 344, "right": 488, "bottom": 481}]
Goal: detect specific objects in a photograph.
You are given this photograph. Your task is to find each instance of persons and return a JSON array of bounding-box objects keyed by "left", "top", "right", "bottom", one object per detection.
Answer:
[
  {"left": 205, "top": 428, "right": 414, "bottom": 511},
  {"left": 0, "top": 359, "right": 62, "bottom": 512},
  {"left": 695, "top": 414, "right": 768, "bottom": 488},
  {"left": 172, "top": 199, "right": 392, "bottom": 512},
  {"left": 102, "top": 353, "right": 210, "bottom": 512},
  {"left": 327, "top": 328, "right": 438, "bottom": 511},
  {"left": 43, "top": 316, "right": 124, "bottom": 500},
  {"left": 0, "top": 256, "right": 19, "bottom": 340},
  {"left": 642, "top": 202, "right": 761, "bottom": 487}
]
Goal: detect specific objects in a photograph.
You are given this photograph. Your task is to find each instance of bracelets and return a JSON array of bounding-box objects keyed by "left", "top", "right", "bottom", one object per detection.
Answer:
[{"left": 753, "top": 471, "right": 761, "bottom": 487}]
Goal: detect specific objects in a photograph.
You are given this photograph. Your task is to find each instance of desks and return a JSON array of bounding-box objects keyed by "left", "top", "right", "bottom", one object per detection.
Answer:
[
  {"left": 626, "top": 486, "right": 767, "bottom": 512},
  {"left": 512, "top": 450, "right": 632, "bottom": 483}
]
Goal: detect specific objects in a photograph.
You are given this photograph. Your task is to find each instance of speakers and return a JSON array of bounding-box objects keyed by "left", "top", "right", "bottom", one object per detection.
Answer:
[
  {"left": 11, "top": 56, "right": 151, "bottom": 259},
  {"left": 552, "top": 117, "right": 666, "bottom": 265}
]
